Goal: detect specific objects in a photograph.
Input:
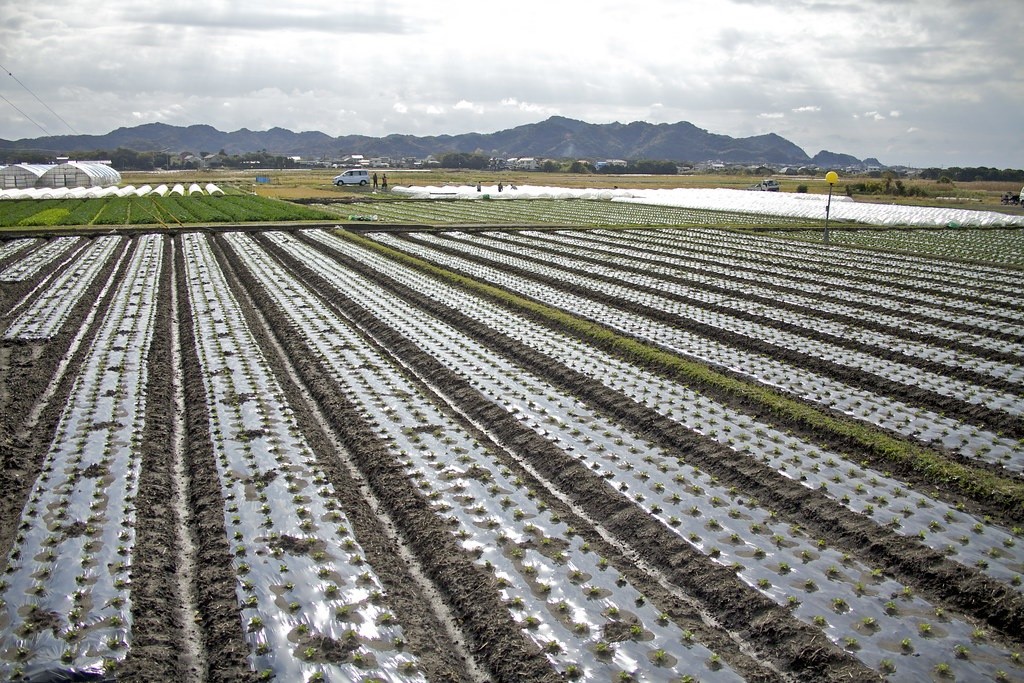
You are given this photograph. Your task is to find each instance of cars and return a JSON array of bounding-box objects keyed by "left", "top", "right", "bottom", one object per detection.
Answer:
[{"left": 1019, "top": 187, "right": 1024, "bottom": 208}]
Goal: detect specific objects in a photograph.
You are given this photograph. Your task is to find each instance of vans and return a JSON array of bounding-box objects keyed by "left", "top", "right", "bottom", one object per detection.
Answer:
[{"left": 331, "top": 168, "right": 370, "bottom": 186}]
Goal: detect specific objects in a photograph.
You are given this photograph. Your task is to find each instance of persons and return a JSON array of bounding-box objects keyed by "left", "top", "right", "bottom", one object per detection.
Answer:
[
  {"left": 382, "top": 174, "right": 388, "bottom": 189},
  {"left": 372, "top": 173, "right": 378, "bottom": 187},
  {"left": 477, "top": 181, "right": 481, "bottom": 192},
  {"left": 510, "top": 184, "right": 518, "bottom": 190},
  {"left": 498, "top": 183, "right": 503, "bottom": 192}
]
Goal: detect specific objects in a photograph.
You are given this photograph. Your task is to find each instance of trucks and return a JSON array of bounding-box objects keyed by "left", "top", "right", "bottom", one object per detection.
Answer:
[{"left": 746, "top": 179, "right": 779, "bottom": 192}]
